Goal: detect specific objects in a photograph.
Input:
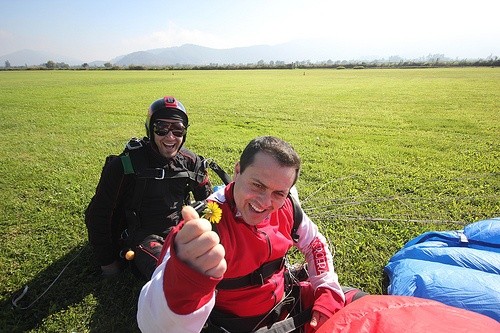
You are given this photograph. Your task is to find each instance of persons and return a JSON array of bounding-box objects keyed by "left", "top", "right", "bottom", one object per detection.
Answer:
[
  {"left": 86, "top": 96, "right": 214, "bottom": 283},
  {"left": 137, "top": 135, "right": 369, "bottom": 333}
]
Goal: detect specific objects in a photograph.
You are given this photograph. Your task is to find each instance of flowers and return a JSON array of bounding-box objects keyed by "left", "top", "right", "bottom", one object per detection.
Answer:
[{"left": 201, "top": 201, "right": 222, "bottom": 224}]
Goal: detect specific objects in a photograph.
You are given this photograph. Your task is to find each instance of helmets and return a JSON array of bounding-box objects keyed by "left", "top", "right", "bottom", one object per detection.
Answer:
[{"left": 145, "top": 97, "right": 189, "bottom": 142}]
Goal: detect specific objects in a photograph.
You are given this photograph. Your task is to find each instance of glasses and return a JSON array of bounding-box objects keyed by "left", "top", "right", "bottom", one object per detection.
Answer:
[{"left": 152, "top": 120, "right": 187, "bottom": 137}]
